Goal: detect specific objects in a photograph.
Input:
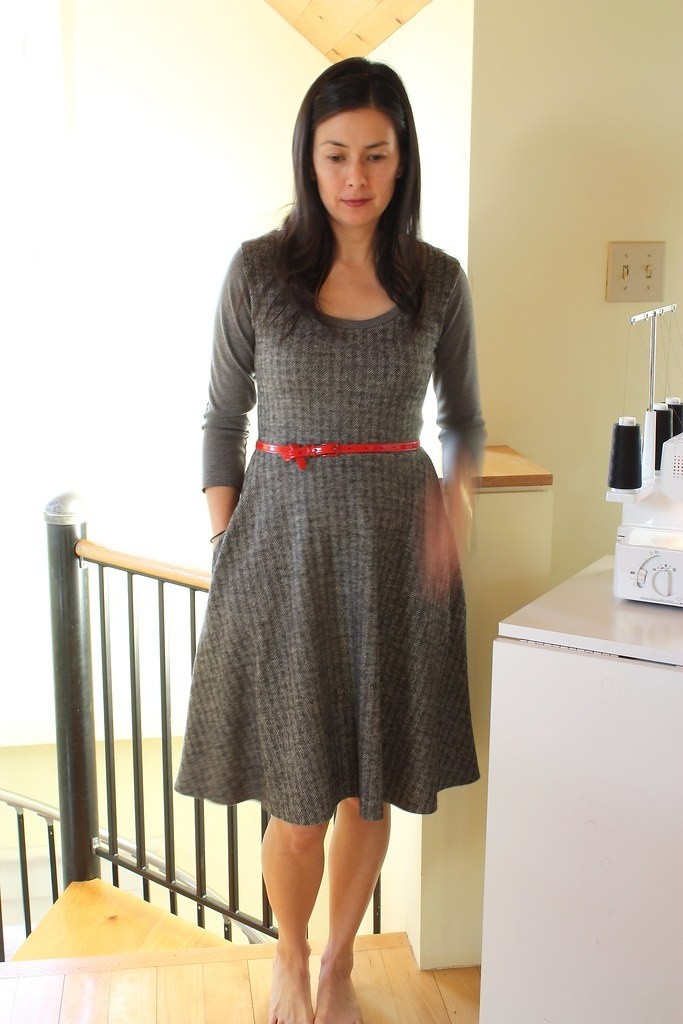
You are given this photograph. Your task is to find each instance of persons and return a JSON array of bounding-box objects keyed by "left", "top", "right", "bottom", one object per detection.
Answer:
[{"left": 176, "top": 56, "right": 483, "bottom": 1024}]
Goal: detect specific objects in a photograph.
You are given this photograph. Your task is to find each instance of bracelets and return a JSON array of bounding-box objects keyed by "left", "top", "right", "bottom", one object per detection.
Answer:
[{"left": 210, "top": 529, "right": 227, "bottom": 543}]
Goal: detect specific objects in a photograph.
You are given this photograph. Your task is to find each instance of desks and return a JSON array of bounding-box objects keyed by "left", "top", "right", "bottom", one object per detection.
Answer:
[{"left": 471, "top": 557, "right": 681, "bottom": 1021}]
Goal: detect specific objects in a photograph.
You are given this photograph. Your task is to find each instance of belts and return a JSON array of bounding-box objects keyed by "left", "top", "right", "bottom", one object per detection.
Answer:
[{"left": 254, "top": 440, "right": 421, "bottom": 469}]
